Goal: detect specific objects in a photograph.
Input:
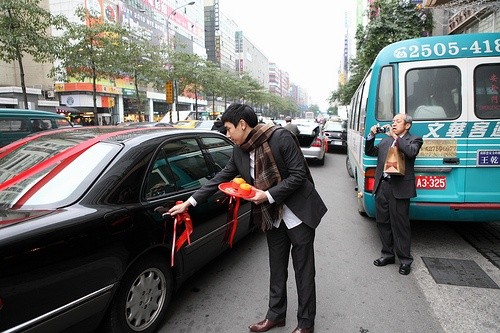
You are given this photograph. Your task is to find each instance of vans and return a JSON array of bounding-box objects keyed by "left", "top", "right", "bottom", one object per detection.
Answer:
[{"left": 0, "top": 106, "right": 75, "bottom": 153}]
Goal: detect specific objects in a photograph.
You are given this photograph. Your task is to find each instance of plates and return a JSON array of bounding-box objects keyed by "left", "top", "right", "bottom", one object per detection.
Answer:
[{"left": 217, "top": 182, "right": 256, "bottom": 198}]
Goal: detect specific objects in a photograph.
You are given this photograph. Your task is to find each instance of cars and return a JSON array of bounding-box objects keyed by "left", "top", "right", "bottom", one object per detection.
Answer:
[
  {"left": 0, "top": 122, "right": 259, "bottom": 333},
  {"left": 116, "top": 109, "right": 348, "bottom": 166}
]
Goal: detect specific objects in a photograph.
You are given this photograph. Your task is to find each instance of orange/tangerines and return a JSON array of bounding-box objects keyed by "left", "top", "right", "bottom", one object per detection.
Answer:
[
  {"left": 233, "top": 178, "right": 244, "bottom": 184},
  {"left": 239, "top": 184, "right": 251, "bottom": 191}
]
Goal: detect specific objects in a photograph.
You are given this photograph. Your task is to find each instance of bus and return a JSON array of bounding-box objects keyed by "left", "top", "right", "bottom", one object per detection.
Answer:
[{"left": 344, "top": 32, "right": 500, "bottom": 226}]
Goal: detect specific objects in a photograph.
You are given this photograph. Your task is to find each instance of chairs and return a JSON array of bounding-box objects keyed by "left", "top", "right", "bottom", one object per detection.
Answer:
[{"left": 412, "top": 103, "right": 445, "bottom": 121}]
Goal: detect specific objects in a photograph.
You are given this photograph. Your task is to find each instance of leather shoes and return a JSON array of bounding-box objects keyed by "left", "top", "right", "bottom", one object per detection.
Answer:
[
  {"left": 398, "top": 264, "right": 410, "bottom": 275},
  {"left": 249, "top": 319, "right": 286, "bottom": 332},
  {"left": 374, "top": 257, "right": 395, "bottom": 265},
  {"left": 292, "top": 327, "right": 314, "bottom": 333}
]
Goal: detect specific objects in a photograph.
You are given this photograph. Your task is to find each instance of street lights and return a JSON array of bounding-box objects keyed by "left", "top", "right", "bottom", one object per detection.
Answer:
[{"left": 165, "top": 1, "right": 196, "bottom": 122}]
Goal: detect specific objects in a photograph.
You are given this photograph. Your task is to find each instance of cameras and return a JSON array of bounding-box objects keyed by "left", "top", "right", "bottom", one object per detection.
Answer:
[{"left": 376, "top": 127, "right": 386, "bottom": 133}]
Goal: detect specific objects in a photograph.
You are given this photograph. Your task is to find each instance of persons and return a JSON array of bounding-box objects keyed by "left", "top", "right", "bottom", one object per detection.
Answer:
[
  {"left": 167, "top": 104, "right": 329, "bottom": 333},
  {"left": 365, "top": 113, "right": 423, "bottom": 275}
]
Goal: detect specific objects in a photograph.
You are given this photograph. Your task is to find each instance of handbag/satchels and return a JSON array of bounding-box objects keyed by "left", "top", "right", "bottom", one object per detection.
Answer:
[{"left": 383, "top": 136, "right": 406, "bottom": 176}]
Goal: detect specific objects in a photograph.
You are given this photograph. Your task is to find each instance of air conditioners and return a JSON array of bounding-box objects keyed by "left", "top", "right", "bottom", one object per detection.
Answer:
[{"left": 48, "top": 90, "right": 55, "bottom": 97}]
